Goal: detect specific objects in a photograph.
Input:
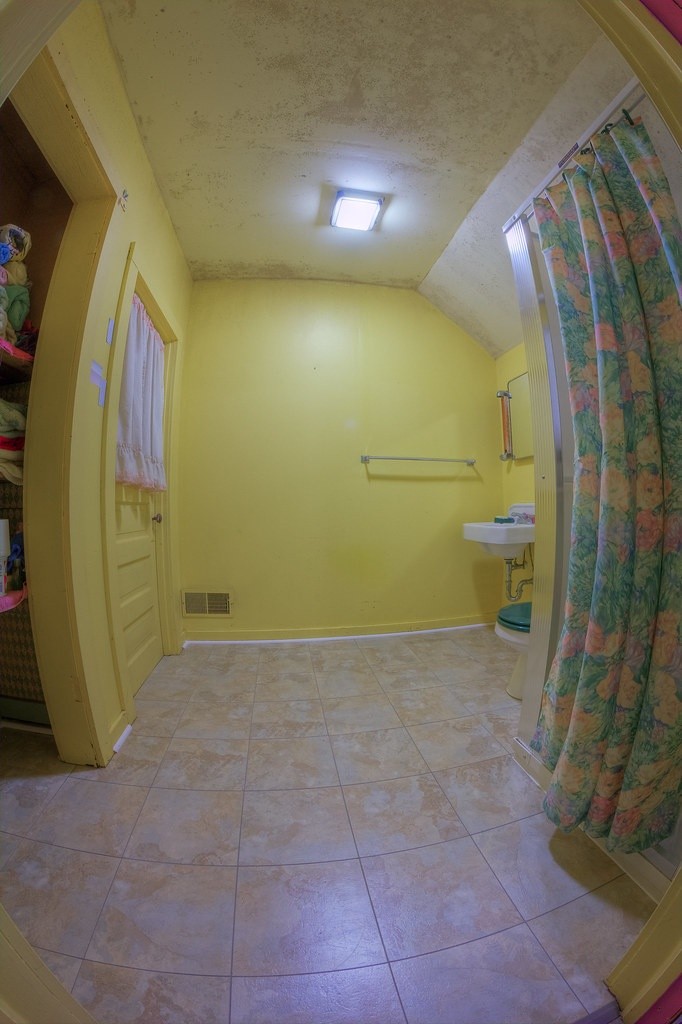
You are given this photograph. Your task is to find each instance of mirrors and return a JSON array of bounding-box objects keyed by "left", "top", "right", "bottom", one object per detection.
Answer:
[{"left": 507, "top": 371, "right": 534, "bottom": 460}]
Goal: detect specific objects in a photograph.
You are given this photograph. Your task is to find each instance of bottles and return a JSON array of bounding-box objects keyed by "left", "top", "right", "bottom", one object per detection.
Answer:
[{"left": 12, "top": 559, "right": 23, "bottom": 590}]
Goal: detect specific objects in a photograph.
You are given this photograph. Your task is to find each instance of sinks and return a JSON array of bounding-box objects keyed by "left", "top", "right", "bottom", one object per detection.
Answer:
[{"left": 462, "top": 503, "right": 535, "bottom": 561}]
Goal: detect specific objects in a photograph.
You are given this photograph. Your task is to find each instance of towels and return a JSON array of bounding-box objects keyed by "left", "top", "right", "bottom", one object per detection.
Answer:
[{"left": 0, "top": 223, "right": 32, "bottom": 488}]
